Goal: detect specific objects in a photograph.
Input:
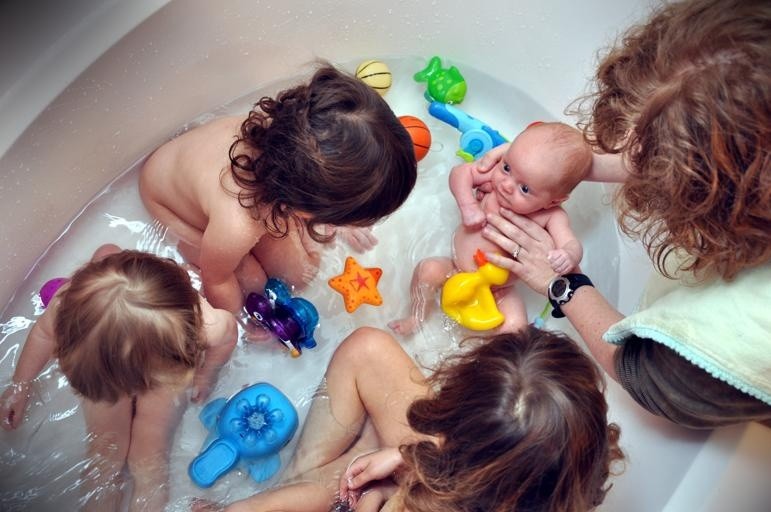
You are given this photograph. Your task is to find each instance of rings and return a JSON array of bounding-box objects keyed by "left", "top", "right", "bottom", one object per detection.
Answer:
[{"left": 513, "top": 245, "right": 522, "bottom": 260}]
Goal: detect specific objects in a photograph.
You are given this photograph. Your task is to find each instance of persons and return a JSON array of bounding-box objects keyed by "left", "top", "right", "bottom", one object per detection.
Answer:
[
  {"left": 189, "top": 327, "right": 622, "bottom": 512},
  {"left": 476, "top": 0, "right": 771, "bottom": 432},
  {"left": 1, "top": 244, "right": 239, "bottom": 512},
  {"left": 137, "top": 61, "right": 419, "bottom": 347},
  {"left": 389, "top": 121, "right": 593, "bottom": 339}
]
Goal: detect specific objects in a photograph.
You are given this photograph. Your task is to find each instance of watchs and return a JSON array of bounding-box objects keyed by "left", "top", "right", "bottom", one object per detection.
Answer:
[{"left": 547, "top": 272, "right": 593, "bottom": 318}]
